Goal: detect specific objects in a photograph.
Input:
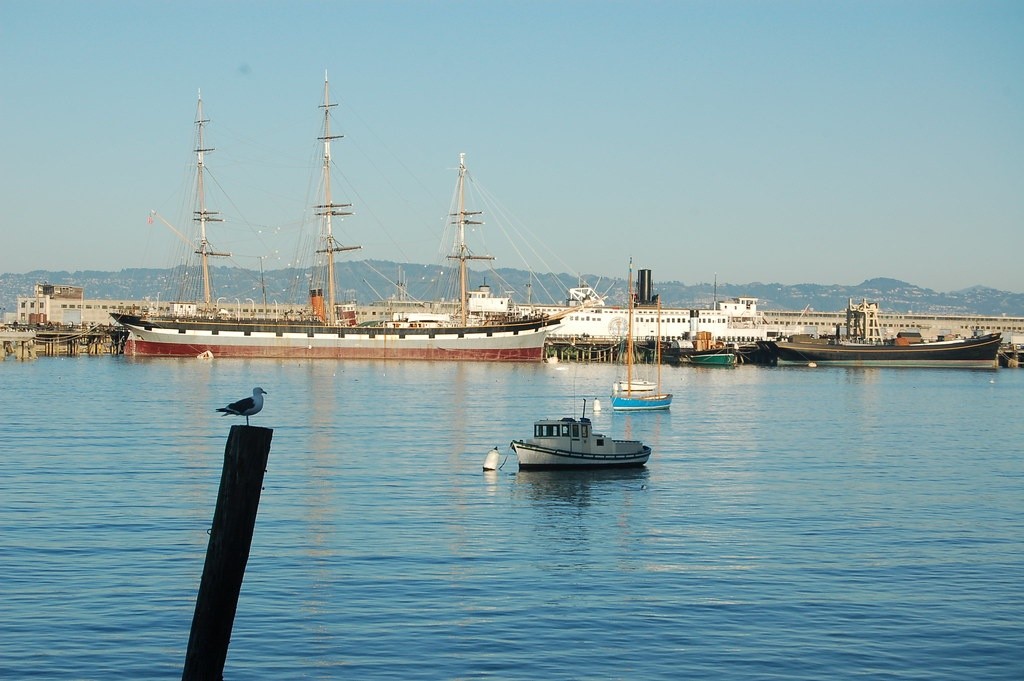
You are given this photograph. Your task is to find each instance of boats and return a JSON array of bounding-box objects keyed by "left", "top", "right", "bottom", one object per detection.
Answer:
[
  {"left": 620, "top": 378, "right": 656, "bottom": 393},
  {"left": 688, "top": 351, "right": 737, "bottom": 366},
  {"left": 481, "top": 398, "right": 652, "bottom": 472},
  {"left": 757, "top": 333, "right": 1004, "bottom": 370}
]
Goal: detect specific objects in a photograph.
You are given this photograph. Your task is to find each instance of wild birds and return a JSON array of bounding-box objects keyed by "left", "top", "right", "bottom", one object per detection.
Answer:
[{"left": 213, "top": 387, "right": 267, "bottom": 425}]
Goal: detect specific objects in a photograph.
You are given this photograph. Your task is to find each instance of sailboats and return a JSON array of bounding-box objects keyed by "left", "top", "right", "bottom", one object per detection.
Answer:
[
  {"left": 105, "top": 67, "right": 566, "bottom": 363},
  {"left": 607, "top": 256, "right": 674, "bottom": 411}
]
